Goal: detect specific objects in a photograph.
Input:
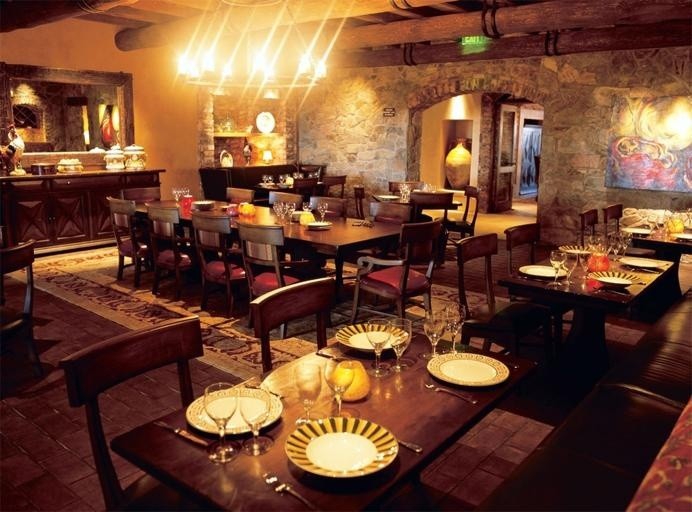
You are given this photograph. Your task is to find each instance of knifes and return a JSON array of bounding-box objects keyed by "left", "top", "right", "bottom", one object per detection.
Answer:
[
  {"left": 152, "top": 418, "right": 208, "bottom": 447},
  {"left": 315, "top": 350, "right": 352, "bottom": 362}
]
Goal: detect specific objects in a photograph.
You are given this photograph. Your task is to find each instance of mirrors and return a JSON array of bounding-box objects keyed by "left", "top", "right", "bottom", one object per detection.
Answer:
[{"left": 0, "top": 62, "right": 134, "bottom": 167}]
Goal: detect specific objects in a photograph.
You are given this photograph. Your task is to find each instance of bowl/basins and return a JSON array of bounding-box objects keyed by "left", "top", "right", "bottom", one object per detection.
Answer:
[
  {"left": 30, "top": 163, "right": 56, "bottom": 176},
  {"left": 192, "top": 200, "right": 216, "bottom": 210}
]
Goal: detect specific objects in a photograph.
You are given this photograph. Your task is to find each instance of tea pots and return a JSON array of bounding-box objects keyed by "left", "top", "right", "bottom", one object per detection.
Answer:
[{"left": 219, "top": 149, "right": 234, "bottom": 168}]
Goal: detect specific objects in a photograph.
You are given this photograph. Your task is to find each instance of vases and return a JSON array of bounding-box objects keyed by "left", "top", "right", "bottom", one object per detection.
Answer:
[{"left": 445, "top": 137, "right": 471, "bottom": 190}]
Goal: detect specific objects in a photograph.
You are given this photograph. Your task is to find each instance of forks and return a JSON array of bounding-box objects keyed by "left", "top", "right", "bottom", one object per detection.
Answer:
[
  {"left": 262, "top": 473, "right": 319, "bottom": 511},
  {"left": 625, "top": 265, "right": 659, "bottom": 274},
  {"left": 425, "top": 382, "right": 478, "bottom": 405}
]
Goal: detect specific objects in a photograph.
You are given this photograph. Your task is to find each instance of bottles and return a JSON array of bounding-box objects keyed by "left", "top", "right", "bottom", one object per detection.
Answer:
[
  {"left": 104, "top": 144, "right": 146, "bottom": 171},
  {"left": 333, "top": 361, "right": 369, "bottom": 402},
  {"left": 226, "top": 201, "right": 256, "bottom": 219}
]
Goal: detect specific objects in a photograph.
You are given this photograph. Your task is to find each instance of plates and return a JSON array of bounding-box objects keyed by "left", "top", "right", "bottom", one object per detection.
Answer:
[
  {"left": 185, "top": 388, "right": 285, "bottom": 436},
  {"left": 519, "top": 265, "right": 567, "bottom": 278},
  {"left": 259, "top": 182, "right": 275, "bottom": 187},
  {"left": 283, "top": 415, "right": 400, "bottom": 479},
  {"left": 378, "top": 193, "right": 400, "bottom": 199},
  {"left": 306, "top": 222, "right": 333, "bottom": 230},
  {"left": 619, "top": 257, "right": 668, "bottom": 269},
  {"left": 219, "top": 205, "right": 230, "bottom": 209},
  {"left": 426, "top": 352, "right": 510, "bottom": 390},
  {"left": 334, "top": 323, "right": 414, "bottom": 354}
]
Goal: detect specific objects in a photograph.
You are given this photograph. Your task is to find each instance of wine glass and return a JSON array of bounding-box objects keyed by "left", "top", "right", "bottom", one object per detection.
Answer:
[
  {"left": 171, "top": 183, "right": 194, "bottom": 209},
  {"left": 204, "top": 382, "right": 237, "bottom": 465},
  {"left": 398, "top": 182, "right": 437, "bottom": 204},
  {"left": 325, "top": 355, "right": 355, "bottom": 420},
  {"left": 290, "top": 360, "right": 328, "bottom": 428},
  {"left": 263, "top": 171, "right": 320, "bottom": 189},
  {"left": 236, "top": 382, "right": 275, "bottom": 453},
  {"left": 365, "top": 301, "right": 467, "bottom": 377},
  {"left": 549, "top": 229, "right": 636, "bottom": 288},
  {"left": 272, "top": 199, "right": 328, "bottom": 226},
  {"left": 622, "top": 207, "right": 692, "bottom": 241}
]
